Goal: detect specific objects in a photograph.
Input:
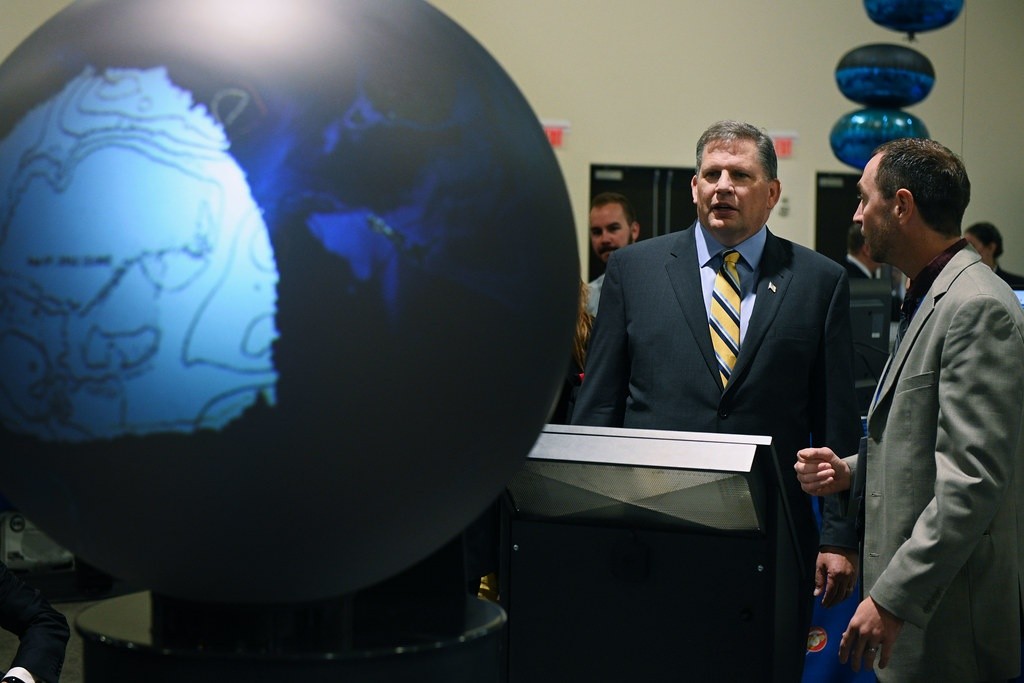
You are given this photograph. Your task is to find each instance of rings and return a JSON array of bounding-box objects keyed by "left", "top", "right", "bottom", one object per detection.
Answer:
[{"left": 867, "top": 647, "right": 878, "bottom": 653}]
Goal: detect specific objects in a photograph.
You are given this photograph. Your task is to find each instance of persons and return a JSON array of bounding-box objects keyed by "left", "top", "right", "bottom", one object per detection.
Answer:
[
  {"left": 584, "top": 192, "right": 639, "bottom": 314},
  {"left": 964, "top": 223, "right": 1024, "bottom": 291},
  {"left": 794, "top": 138, "right": 1023, "bottom": 683},
  {"left": 836, "top": 224, "right": 903, "bottom": 322},
  {"left": 0, "top": 559, "right": 71, "bottom": 683},
  {"left": 551, "top": 278, "right": 595, "bottom": 423},
  {"left": 569, "top": 121, "right": 864, "bottom": 683}
]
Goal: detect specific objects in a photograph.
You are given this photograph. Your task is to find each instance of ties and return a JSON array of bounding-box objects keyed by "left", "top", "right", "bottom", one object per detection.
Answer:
[
  {"left": 893, "top": 283, "right": 917, "bottom": 366},
  {"left": 709, "top": 248, "right": 741, "bottom": 390}
]
